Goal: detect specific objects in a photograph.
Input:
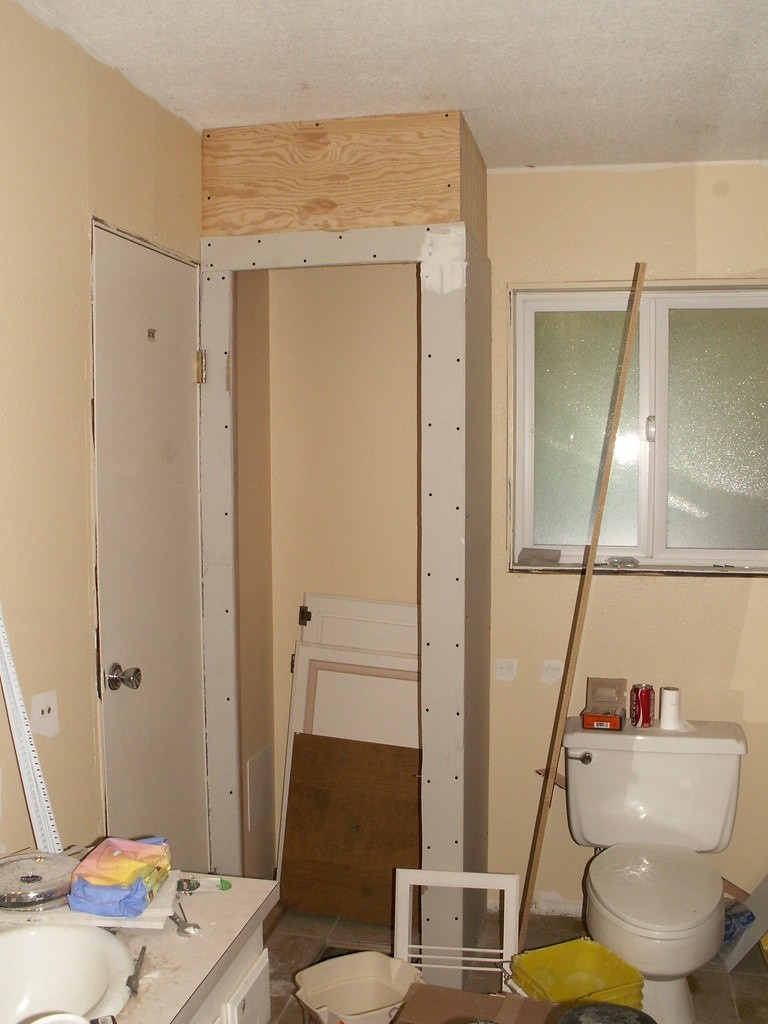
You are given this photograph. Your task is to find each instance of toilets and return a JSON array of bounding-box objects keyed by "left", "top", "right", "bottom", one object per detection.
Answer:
[{"left": 561, "top": 719, "right": 749, "bottom": 1024}]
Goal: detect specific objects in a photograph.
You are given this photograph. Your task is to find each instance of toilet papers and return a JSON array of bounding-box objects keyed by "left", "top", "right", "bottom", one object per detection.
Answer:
[{"left": 658, "top": 687, "right": 681, "bottom": 730}]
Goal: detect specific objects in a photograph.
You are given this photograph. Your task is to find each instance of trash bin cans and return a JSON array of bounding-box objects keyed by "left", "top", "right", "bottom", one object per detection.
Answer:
[{"left": 294, "top": 951, "right": 425, "bottom": 1024}]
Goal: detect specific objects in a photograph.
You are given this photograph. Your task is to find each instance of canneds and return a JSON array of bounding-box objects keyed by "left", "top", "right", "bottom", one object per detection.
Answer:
[{"left": 630, "top": 683, "right": 655, "bottom": 729}]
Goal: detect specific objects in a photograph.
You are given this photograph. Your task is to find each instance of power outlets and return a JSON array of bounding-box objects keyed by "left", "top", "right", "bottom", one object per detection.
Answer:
[{"left": 29, "top": 690, "right": 59, "bottom": 737}]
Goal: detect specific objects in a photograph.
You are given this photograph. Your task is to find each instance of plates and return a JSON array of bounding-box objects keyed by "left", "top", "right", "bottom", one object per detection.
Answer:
[
  {"left": 556, "top": 1004, "right": 657, "bottom": 1024},
  {"left": 294, "top": 951, "right": 427, "bottom": 1024}
]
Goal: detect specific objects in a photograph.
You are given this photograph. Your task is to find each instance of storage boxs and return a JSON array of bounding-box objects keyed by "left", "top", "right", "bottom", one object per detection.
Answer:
[
  {"left": 295, "top": 951, "right": 427, "bottom": 1024},
  {"left": 580, "top": 677, "right": 627, "bottom": 730}
]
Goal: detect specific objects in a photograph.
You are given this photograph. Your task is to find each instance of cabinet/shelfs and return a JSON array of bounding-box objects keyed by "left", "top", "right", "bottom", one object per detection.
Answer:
[{"left": 187, "top": 920, "right": 272, "bottom": 1024}]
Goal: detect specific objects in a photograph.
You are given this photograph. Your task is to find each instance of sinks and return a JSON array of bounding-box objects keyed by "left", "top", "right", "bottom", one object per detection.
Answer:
[{"left": 0, "top": 919, "right": 136, "bottom": 1024}]
